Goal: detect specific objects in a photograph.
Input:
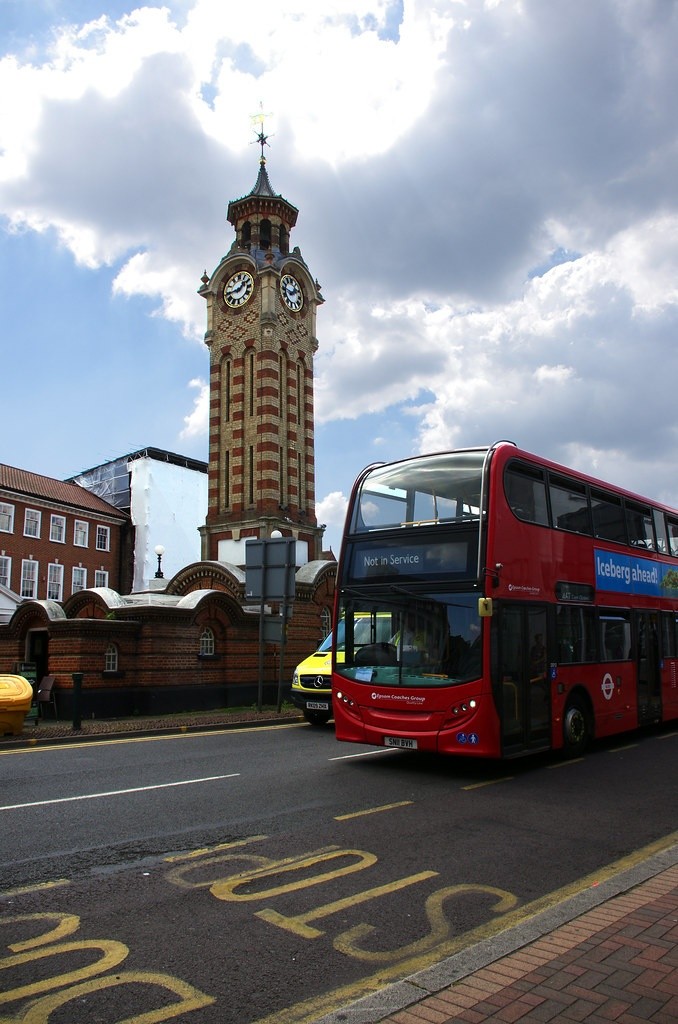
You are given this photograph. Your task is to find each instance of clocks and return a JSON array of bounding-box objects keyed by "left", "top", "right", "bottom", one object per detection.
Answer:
[
  {"left": 223, "top": 271, "right": 255, "bottom": 308},
  {"left": 279, "top": 274, "right": 304, "bottom": 312}
]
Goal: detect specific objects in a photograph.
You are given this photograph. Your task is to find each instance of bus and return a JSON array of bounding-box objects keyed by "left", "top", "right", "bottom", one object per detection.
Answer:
[{"left": 329, "top": 441, "right": 678, "bottom": 764}]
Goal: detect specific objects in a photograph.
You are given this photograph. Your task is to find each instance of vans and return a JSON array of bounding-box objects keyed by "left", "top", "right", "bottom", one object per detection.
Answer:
[{"left": 291, "top": 611, "right": 425, "bottom": 726}]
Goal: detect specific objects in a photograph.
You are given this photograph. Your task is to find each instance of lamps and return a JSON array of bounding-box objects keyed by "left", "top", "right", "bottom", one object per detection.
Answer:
[
  {"left": 153, "top": 545, "right": 165, "bottom": 577},
  {"left": 270, "top": 530, "right": 282, "bottom": 538}
]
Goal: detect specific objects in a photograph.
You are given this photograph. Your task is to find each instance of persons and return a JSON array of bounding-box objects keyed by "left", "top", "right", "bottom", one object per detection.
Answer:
[{"left": 386, "top": 616, "right": 433, "bottom": 651}]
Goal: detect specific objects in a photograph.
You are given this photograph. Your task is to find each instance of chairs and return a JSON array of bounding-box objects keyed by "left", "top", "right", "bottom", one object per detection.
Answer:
[{"left": 32, "top": 676, "right": 57, "bottom": 722}]
